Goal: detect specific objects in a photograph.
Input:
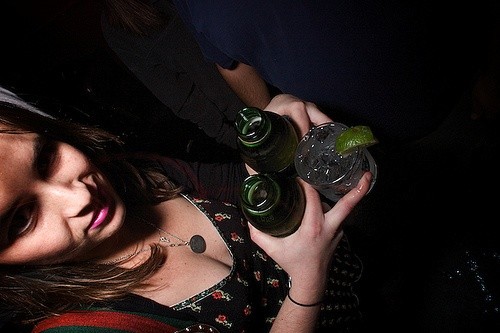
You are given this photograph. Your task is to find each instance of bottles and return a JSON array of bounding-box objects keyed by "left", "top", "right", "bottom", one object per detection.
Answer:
[
  {"left": 235, "top": 106, "right": 301, "bottom": 178},
  {"left": 239, "top": 171, "right": 306, "bottom": 238}
]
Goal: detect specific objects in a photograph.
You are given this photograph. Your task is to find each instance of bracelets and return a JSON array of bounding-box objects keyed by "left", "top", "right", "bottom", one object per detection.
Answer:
[{"left": 287, "top": 289, "right": 325, "bottom": 307}]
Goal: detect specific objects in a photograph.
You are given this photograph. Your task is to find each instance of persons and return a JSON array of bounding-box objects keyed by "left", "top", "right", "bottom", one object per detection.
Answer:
[
  {"left": 98, "top": 1, "right": 500, "bottom": 171},
  {"left": 0, "top": 93, "right": 405, "bottom": 333}
]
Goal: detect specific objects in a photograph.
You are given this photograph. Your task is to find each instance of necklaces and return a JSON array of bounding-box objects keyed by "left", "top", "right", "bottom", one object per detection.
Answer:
[{"left": 103, "top": 218, "right": 206, "bottom": 264}]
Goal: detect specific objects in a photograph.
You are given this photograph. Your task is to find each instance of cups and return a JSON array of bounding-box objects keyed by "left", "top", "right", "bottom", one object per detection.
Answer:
[{"left": 294, "top": 122, "right": 377, "bottom": 203}]
